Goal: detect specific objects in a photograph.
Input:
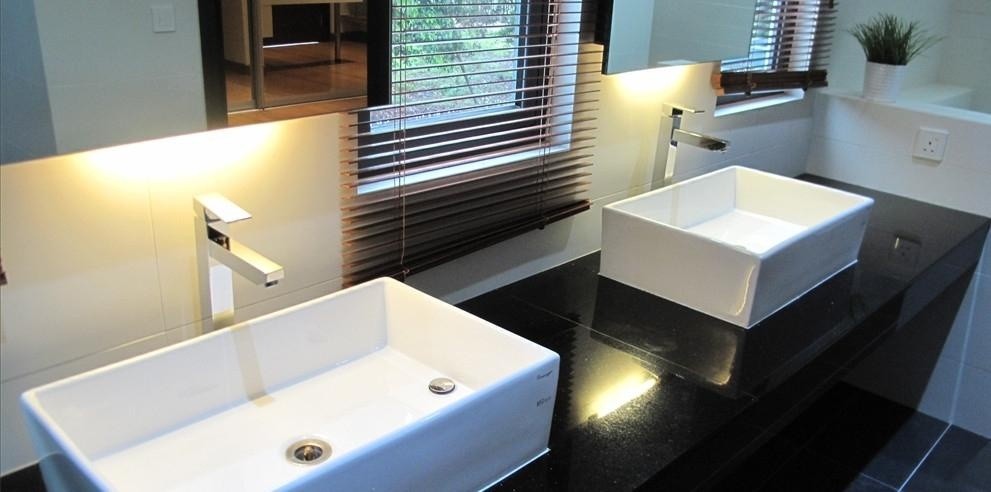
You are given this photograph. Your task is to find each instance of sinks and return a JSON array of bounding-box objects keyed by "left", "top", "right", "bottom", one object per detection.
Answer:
[
  {"left": 20, "top": 276, "right": 556, "bottom": 492},
  {"left": 595, "top": 163, "right": 874, "bottom": 329}
]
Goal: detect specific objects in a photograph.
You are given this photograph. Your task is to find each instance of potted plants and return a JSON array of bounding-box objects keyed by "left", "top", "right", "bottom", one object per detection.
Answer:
[{"left": 842, "top": 7, "right": 951, "bottom": 100}]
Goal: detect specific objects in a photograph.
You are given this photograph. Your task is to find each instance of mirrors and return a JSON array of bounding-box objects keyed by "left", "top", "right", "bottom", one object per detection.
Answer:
[
  {"left": 599, "top": 0, "right": 757, "bottom": 77},
  {"left": 197, "top": 1, "right": 392, "bottom": 134}
]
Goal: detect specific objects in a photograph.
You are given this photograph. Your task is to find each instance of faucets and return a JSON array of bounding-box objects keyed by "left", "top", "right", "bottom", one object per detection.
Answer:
[
  {"left": 648, "top": 100, "right": 729, "bottom": 181},
  {"left": 191, "top": 190, "right": 285, "bottom": 320}
]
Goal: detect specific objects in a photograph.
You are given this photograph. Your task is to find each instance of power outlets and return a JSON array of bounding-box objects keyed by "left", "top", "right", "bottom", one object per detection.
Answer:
[
  {"left": 886, "top": 236, "right": 921, "bottom": 278},
  {"left": 913, "top": 127, "right": 950, "bottom": 163}
]
